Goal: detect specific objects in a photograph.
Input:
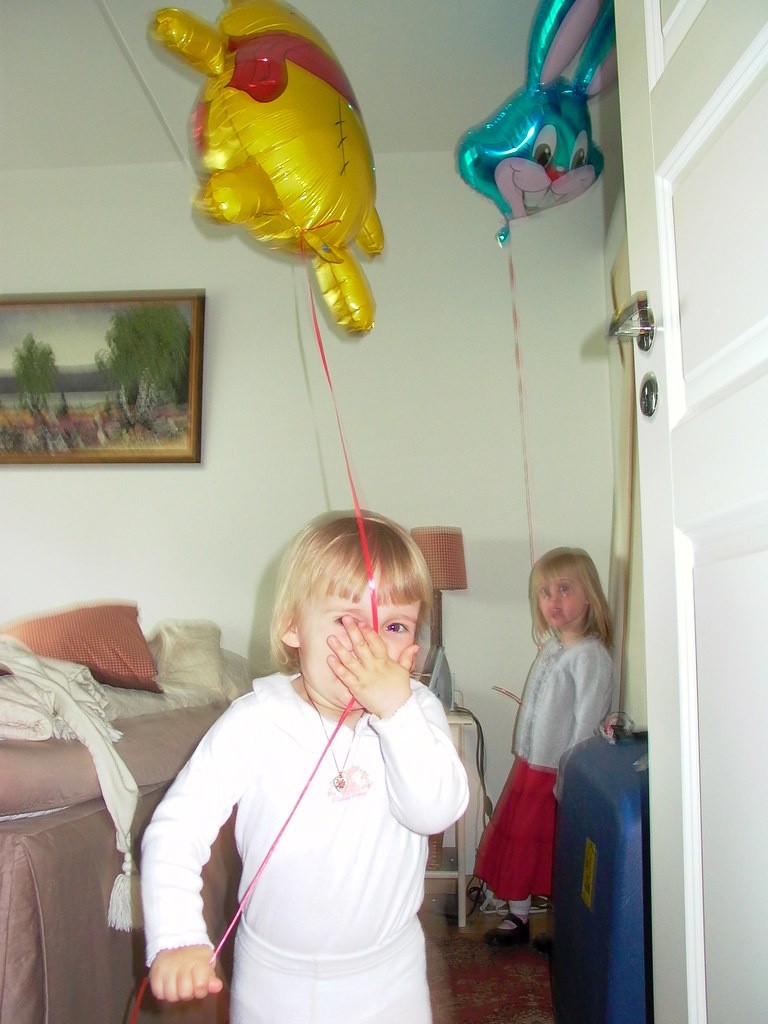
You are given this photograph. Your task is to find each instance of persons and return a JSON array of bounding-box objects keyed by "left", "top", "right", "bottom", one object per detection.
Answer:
[
  {"left": 475, "top": 548, "right": 613, "bottom": 947},
  {"left": 139, "top": 515, "right": 468, "bottom": 1024}
]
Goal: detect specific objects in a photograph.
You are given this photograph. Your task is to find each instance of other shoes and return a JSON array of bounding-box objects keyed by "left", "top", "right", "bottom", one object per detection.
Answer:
[
  {"left": 484, "top": 911, "right": 529, "bottom": 947},
  {"left": 533, "top": 934, "right": 553, "bottom": 955}
]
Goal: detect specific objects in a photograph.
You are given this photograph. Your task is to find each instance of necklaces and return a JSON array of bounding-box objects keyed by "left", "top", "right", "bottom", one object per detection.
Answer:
[{"left": 305, "top": 689, "right": 364, "bottom": 792}]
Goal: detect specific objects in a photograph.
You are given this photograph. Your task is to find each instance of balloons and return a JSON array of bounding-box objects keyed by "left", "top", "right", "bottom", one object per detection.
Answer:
[
  {"left": 152, "top": 0, "right": 384, "bottom": 334},
  {"left": 458, "top": 0, "right": 617, "bottom": 242}
]
